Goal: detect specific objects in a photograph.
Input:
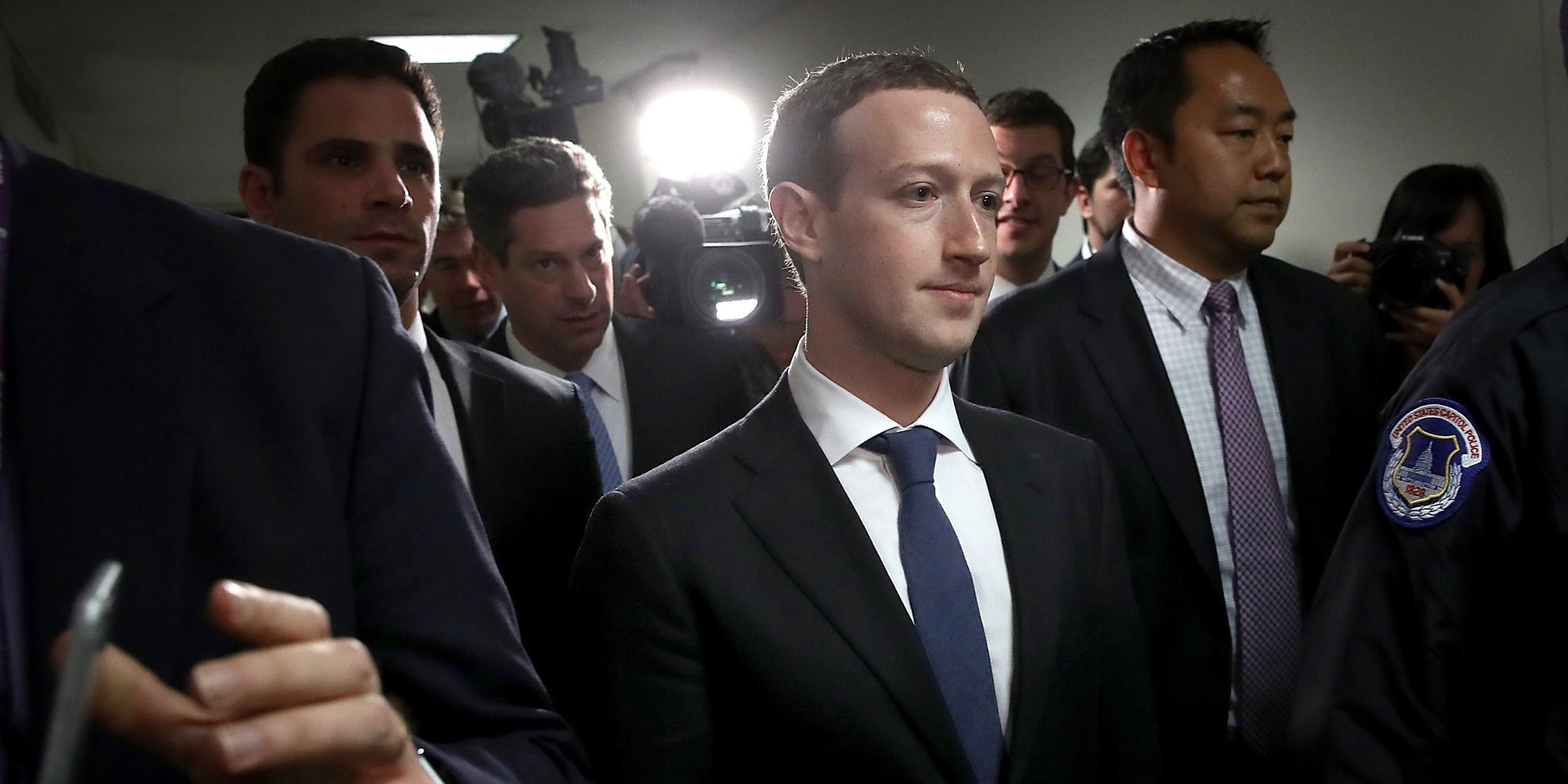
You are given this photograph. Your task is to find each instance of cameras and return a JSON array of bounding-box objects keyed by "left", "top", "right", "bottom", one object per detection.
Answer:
[{"left": 1353, "top": 235, "right": 1456, "bottom": 333}]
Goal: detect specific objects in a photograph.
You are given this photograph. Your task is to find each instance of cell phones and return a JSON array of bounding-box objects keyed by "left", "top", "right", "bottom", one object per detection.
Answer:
[{"left": 36, "top": 562, "right": 120, "bottom": 784}]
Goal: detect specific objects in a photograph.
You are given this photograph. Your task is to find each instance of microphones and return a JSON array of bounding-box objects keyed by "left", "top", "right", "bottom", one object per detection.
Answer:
[{"left": 631, "top": 195, "right": 708, "bottom": 266}]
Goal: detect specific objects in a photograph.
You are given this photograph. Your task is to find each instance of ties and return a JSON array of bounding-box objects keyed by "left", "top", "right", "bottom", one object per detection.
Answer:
[
  {"left": 564, "top": 373, "right": 621, "bottom": 497},
  {"left": 859, "top": 425, "right": 1006, "bottom": 784},
  {"left": 1204, "top": 283, "right": 1304, "bottom": 777}
]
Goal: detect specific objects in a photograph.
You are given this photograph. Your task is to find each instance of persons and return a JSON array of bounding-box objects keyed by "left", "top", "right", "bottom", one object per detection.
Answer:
[
  {"left": 1, "top": 89, "right": 583, "bottom": 784},
  {"left": 1329, "top": 158, "right": 1514, "bottom": 370},
  {"left": 423, "top": 89, "right": 1124, "bottom": 583},
  {"left": 236, "top": 37, "right": 603, "bottom": 594},
  {"left": 968, "top": 18, "right": 1423, "bottom": 784},
  {"left": 1249, "top": 0, "right": 1568, "bottom": 784},
  {"left": 556, "top": 53, "right": 1138, "bottom": 784}
]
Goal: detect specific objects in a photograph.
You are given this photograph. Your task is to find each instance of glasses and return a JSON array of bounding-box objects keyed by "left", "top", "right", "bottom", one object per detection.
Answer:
[{"left": 999, "top": 163, "right": 1072, "bottom": 192}]
